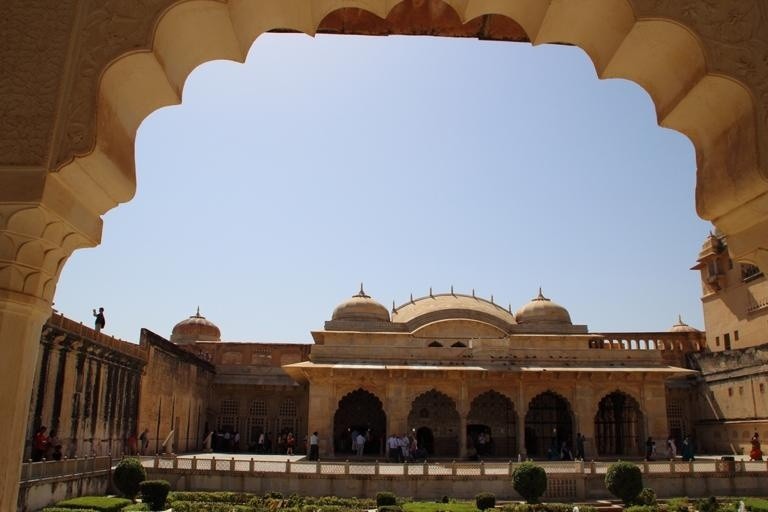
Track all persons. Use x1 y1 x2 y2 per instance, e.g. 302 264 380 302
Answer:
32 426 49 461
128 431 138 455
380 431 386 456
257 430 297 456
387 431 428 463
574 433 585 461
647 433 696 461
43 429 62 460
140 428 151 455
467 430 491 461
749 431 762 461
356 432 365 456
93 307 105 333
304 433 308 455
352 429 359 455
212 429 241 453
309 431 320 461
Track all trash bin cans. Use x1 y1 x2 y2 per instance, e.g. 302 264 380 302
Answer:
721 457 735 473
